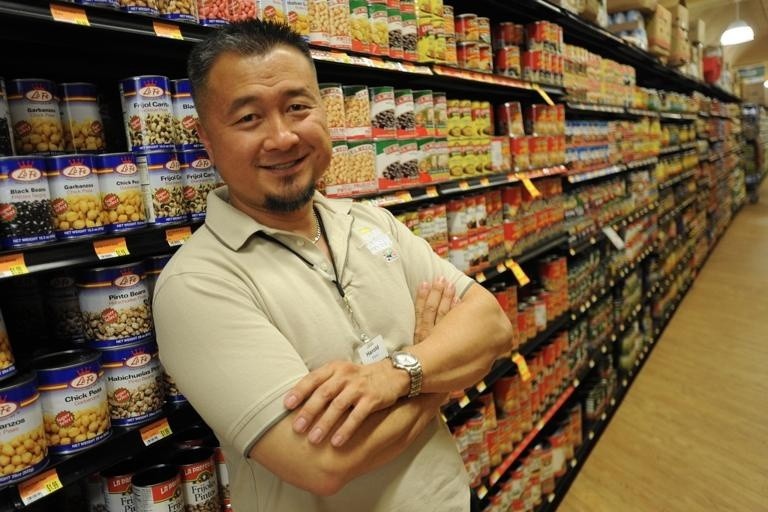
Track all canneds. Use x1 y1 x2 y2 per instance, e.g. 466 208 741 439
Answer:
1 0 565 512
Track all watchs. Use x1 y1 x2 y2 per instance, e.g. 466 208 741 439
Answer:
382 349 424 400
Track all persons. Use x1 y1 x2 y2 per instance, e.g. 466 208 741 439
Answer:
150 15 515 511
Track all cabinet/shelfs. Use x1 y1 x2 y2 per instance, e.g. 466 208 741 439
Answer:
0 0 745 512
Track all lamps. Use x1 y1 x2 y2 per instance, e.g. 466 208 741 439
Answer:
716 0 756 48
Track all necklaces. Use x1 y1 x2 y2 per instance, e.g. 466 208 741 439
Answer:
306 207 322 245
